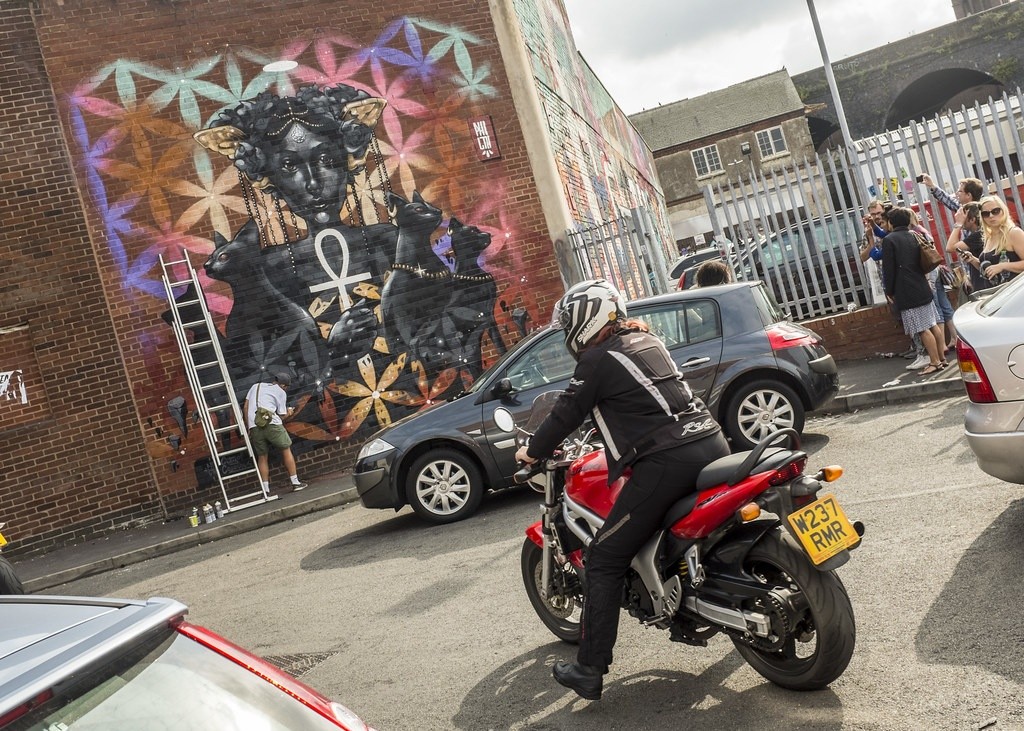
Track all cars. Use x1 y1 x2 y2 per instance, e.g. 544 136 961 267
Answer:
351 281 858 524
950 270 1024 482
669 239 789 294
0 585 376 731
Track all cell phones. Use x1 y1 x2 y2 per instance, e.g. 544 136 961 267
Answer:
916 175 923 183
956 247 969 259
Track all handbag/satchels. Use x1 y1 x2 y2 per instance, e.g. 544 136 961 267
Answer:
939 266 955 285
909 229 944 275
253 405 273 428
859 255 889 310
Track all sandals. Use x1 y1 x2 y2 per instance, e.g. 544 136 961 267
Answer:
939 359 949 367
917 363 944 376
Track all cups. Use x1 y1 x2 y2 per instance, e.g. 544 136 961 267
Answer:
189 516 198 527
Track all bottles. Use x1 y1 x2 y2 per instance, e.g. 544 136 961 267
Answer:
214 501 224 518
202 505 213 524
206 503 217 522
192 506 201 524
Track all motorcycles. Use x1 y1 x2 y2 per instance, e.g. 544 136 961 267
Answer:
482 386 875 693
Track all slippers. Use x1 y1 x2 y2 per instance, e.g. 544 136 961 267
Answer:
943 349 951 355
947 344 956 352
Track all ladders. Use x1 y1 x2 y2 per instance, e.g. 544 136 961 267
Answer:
158 248 282 517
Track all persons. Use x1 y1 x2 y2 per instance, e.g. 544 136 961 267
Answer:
514 276 730 701
859 200 960 376
242 372 309 497
678 261 731 341
917 172 1024 293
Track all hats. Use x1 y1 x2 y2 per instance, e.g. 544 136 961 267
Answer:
275 371 292 385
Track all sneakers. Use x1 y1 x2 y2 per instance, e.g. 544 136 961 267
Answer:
261 491 278 499
552 661 608 701
905 354 932 371
290 482 308 492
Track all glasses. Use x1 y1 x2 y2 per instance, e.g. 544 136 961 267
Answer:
871 211 881 218
981 207 1002 218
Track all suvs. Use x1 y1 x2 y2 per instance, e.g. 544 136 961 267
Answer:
678 201 937 326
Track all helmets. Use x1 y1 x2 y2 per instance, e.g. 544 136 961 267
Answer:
557 279 628 362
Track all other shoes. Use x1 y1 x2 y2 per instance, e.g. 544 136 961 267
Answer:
904 351 918 360
898 348 912 357
